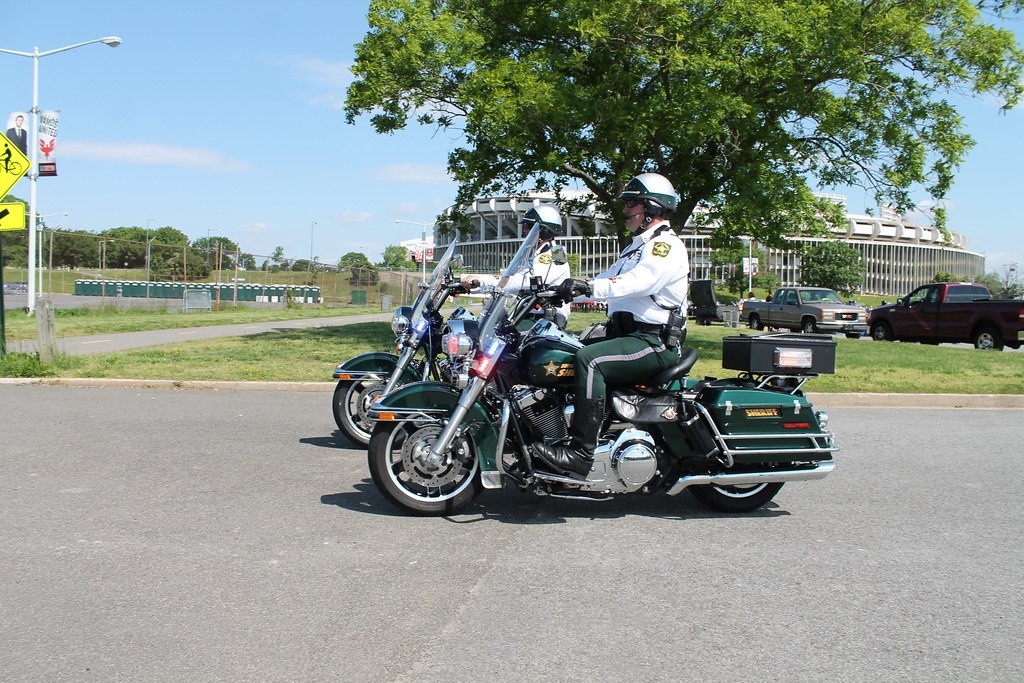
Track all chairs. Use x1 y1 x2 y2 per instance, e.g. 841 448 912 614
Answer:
788 293 796 300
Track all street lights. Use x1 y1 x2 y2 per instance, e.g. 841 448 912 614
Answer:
98 240 114 273
207 228 221 264
0 37 121 320
146 219 159 273
395 220 454 283
25 213 68 296
311 221 318 284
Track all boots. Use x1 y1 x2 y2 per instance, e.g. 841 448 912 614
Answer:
530 397 606 483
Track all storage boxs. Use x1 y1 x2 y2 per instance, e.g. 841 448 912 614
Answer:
721 336 838 375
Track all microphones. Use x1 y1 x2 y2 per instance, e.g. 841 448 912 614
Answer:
522 234 527 238
623 210 646 221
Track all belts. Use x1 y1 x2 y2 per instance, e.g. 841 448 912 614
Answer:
632 321 686 340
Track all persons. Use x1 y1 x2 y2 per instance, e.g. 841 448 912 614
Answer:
548 173 690 481
5 114 27 158
460 204 571 332
735 290 772 329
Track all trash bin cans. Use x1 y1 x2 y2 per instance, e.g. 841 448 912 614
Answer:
689 280 740 329
381 294 394 312
352 291 366 305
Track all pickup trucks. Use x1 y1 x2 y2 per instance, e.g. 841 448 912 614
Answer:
742 288 869 339
866 282 1024 352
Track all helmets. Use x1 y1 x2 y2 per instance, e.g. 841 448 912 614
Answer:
617 173 676 213
516 205 562 236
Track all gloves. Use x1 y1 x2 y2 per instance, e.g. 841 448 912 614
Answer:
555 278 592 304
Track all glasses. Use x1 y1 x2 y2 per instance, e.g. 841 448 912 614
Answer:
524 223 534 230
624 199 647 207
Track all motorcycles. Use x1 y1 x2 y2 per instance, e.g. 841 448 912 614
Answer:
366 223 840 515
333 239 606 449
